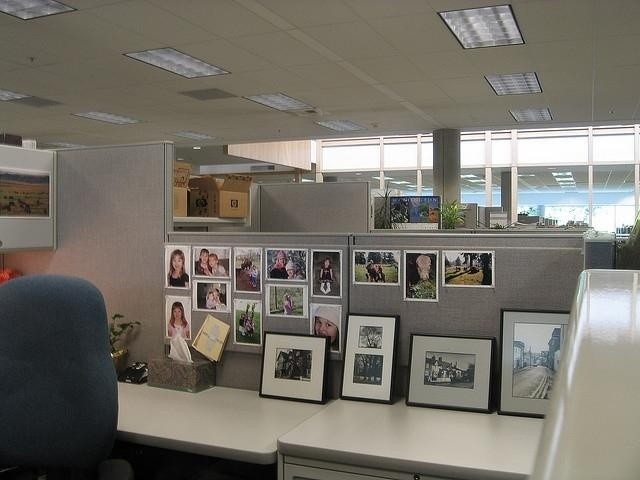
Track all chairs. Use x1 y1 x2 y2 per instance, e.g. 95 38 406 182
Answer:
0 275 119 480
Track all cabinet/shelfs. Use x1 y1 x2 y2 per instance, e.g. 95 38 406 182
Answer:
276 398 543 480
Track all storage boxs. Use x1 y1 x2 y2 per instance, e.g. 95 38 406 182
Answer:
173 161 192 216
188 173 253 219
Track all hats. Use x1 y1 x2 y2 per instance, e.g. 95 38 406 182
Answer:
276 250 297 272
315 307 339 330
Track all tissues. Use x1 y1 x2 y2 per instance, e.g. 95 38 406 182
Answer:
147 325 217 393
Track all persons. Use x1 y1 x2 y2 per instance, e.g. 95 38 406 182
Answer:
167 249 385 377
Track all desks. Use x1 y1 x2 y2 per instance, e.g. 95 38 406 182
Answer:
115 379 336 465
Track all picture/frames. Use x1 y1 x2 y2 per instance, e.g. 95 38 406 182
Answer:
497 308 569 419
260 331 331 404
0 167 51 219
352 249 495 302
164 244 341 354
340 313 399 404
405 333 495 414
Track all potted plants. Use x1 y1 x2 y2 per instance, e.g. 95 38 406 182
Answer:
108 314 141 379
